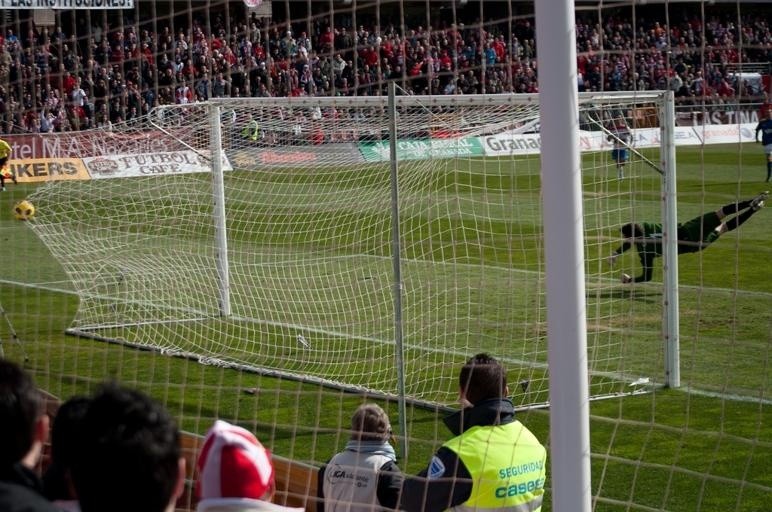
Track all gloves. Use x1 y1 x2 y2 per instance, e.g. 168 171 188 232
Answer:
607 251 619 266
621 274 632 284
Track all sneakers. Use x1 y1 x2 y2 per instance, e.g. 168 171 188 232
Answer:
764 177 771 183
616 174 625 179
750 190 769 213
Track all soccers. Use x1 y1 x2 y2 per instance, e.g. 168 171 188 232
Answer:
13 201 34 221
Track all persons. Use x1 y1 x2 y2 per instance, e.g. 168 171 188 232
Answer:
0 140 20 192
602 186 771 284
33 368 188 511
187 416 308 509
316 399 400 512
1 22 772 135
0 351 62 511
394 347 547 511
604 109 634 181
752 108 771 184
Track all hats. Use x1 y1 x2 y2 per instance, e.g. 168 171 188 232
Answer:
193 419 276 501
